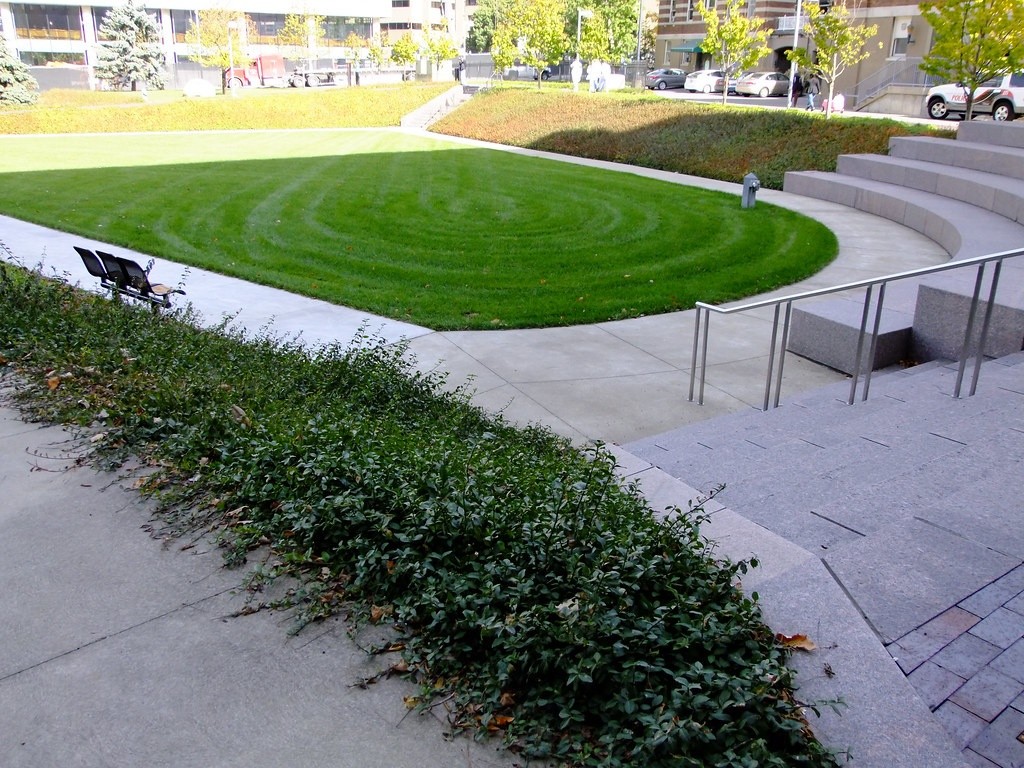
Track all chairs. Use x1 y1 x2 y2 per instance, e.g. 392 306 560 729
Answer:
74 244 185 315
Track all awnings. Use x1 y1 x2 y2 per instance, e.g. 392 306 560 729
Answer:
670 40 712 54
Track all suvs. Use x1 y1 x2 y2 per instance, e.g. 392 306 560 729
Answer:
925 69 1024 122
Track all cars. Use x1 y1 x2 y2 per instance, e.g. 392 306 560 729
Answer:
502 57 552 81
735 72 791 98
685 69 733 94
645 68 689 90
715 71 753 96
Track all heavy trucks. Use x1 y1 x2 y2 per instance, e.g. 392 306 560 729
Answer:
223 52 336 89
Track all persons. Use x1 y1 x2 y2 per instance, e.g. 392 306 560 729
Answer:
791 74 822 111
458 58 467 84
570 56 583 95
831 91 846 111
585 58 611 92
